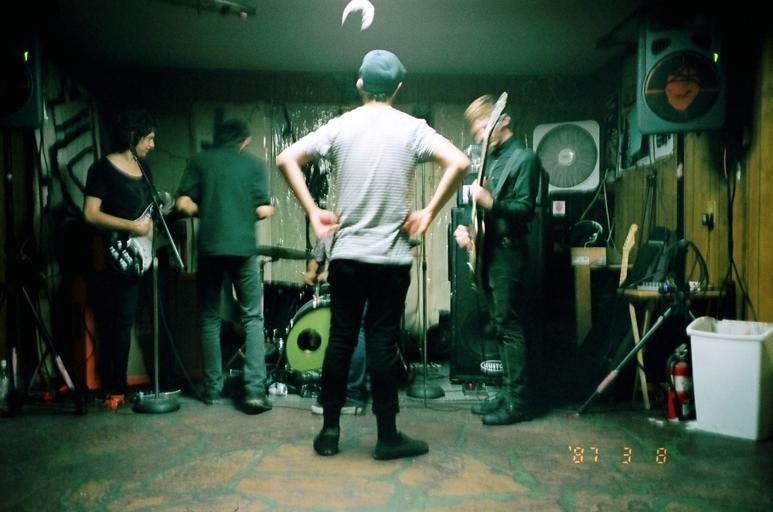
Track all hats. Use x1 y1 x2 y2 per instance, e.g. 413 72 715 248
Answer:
359 50 406 93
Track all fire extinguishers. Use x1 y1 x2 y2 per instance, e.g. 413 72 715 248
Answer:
665 343 692 420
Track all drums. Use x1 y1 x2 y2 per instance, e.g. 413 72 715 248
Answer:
262 280 295 338
280 281 366 389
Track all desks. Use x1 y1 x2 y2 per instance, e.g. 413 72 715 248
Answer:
615 287 726 411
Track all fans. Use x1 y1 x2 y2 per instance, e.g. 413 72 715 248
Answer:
533 120 602 193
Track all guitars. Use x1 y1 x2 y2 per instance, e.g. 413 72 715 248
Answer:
467 90 508 288
109 196 181 281
616 223 640 290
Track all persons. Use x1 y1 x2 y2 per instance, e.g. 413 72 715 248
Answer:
274 46 474 462
451 92 543 426
173 116 277 411
301 234 371 417
80 104 197 412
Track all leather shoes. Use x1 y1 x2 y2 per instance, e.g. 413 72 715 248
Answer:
472 394 531 425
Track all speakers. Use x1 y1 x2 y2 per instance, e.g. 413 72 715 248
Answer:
0 0 45 130
638 0 728 135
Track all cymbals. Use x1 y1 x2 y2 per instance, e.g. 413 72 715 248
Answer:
256 244 316 261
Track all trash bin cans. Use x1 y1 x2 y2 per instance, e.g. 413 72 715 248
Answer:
686 316 773 441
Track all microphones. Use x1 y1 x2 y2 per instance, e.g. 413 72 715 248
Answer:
128 128 138 154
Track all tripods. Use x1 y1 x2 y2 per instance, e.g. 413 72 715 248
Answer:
578 140 698 416
0 131 87 416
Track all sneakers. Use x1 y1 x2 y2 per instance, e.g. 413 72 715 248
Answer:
244 392 272 409
314 433 338 455
374 432 429 460
203 394 219 404
